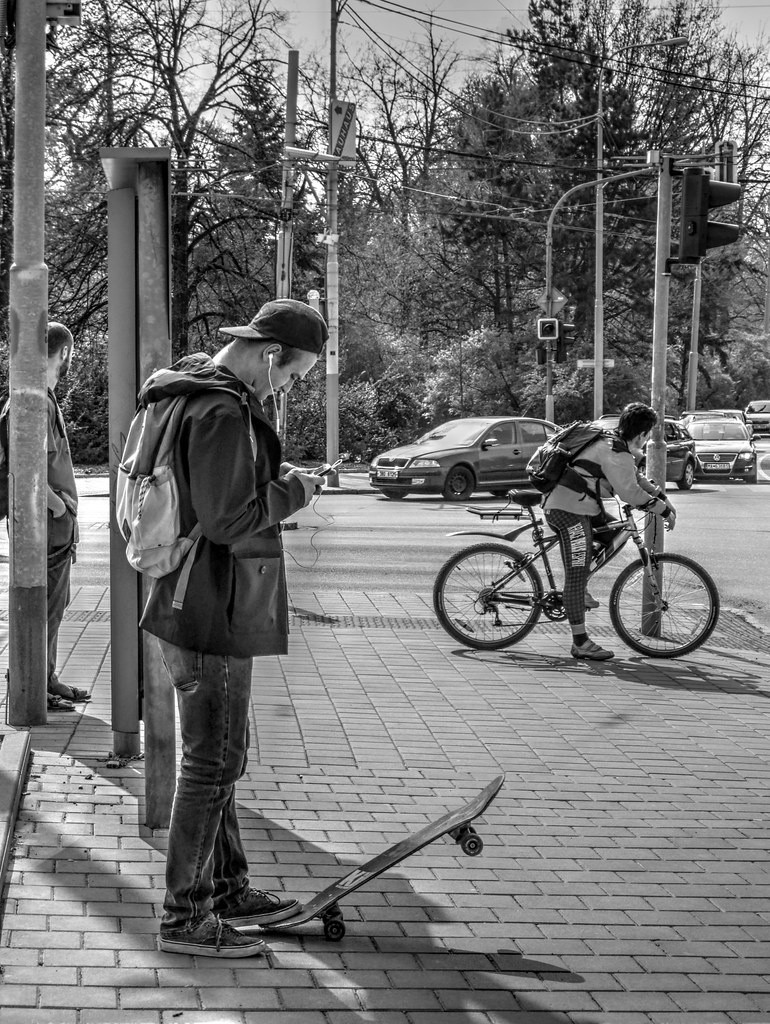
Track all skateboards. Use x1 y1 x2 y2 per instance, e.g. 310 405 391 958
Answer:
265 774 507 942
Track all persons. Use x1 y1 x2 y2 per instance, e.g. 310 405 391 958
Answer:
115 299 326 958
0 322 90 712
542 402 675 660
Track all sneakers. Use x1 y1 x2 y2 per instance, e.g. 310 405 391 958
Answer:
216 887 303 926
584 589 599 608
157 911 265 958
571 640 614 660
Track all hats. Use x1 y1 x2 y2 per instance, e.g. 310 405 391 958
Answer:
218 298 329 355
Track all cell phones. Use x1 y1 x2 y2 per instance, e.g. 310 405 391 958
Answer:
309 464 331 476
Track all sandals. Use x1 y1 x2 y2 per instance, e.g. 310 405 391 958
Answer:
47 691 76 711
48 681 91 703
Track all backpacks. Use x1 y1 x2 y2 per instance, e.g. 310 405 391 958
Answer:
525 421 632 501
0 388 65 521
115 380 257 610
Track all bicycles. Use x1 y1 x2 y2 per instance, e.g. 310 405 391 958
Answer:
432 489 720 660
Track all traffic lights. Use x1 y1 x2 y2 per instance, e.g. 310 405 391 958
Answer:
538 318 559 340
681 166 741 259
556 321 575 363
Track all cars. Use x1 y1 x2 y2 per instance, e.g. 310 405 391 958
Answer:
680 409 754 441
368 417 566 501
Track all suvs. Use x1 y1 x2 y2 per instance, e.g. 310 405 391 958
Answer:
685 416 761 484
590 415 697 490
745 400 770 430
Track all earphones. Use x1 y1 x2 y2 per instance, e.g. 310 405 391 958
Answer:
268 354 274 368
